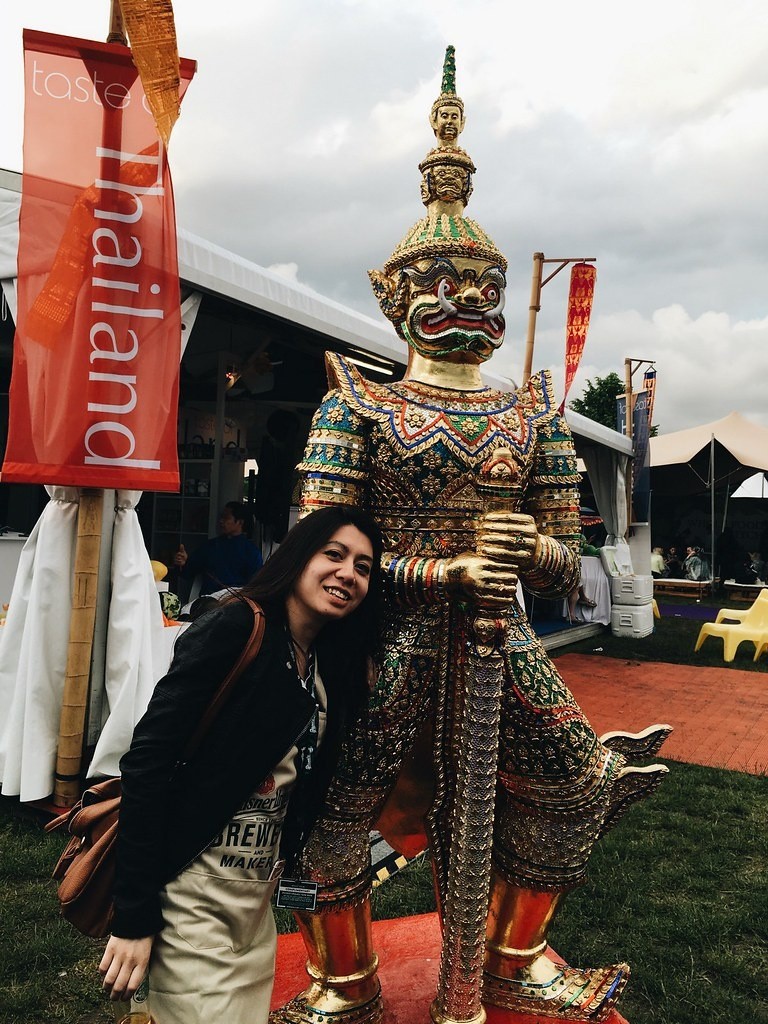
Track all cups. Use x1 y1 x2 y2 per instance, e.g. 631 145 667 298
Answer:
197 478 210 497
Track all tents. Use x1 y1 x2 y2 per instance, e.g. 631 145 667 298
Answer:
575 410 768 578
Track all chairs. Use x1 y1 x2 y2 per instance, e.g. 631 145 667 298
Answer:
715 588 768 652
695 599 768 662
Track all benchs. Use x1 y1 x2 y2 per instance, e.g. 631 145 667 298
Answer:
722 580 768 601
654 574 720 599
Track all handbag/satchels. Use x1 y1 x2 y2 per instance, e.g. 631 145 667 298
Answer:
43 775 122 939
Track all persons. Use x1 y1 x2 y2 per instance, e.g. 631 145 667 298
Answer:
267 46 630 1024
99 503 379 1024
651 546 711 593
175 501 263 596
744 553 766 583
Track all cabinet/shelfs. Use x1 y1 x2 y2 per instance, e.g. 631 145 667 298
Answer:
152 458 245 601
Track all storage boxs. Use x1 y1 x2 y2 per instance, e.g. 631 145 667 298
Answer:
612 605 654 638
600 546 653 605
